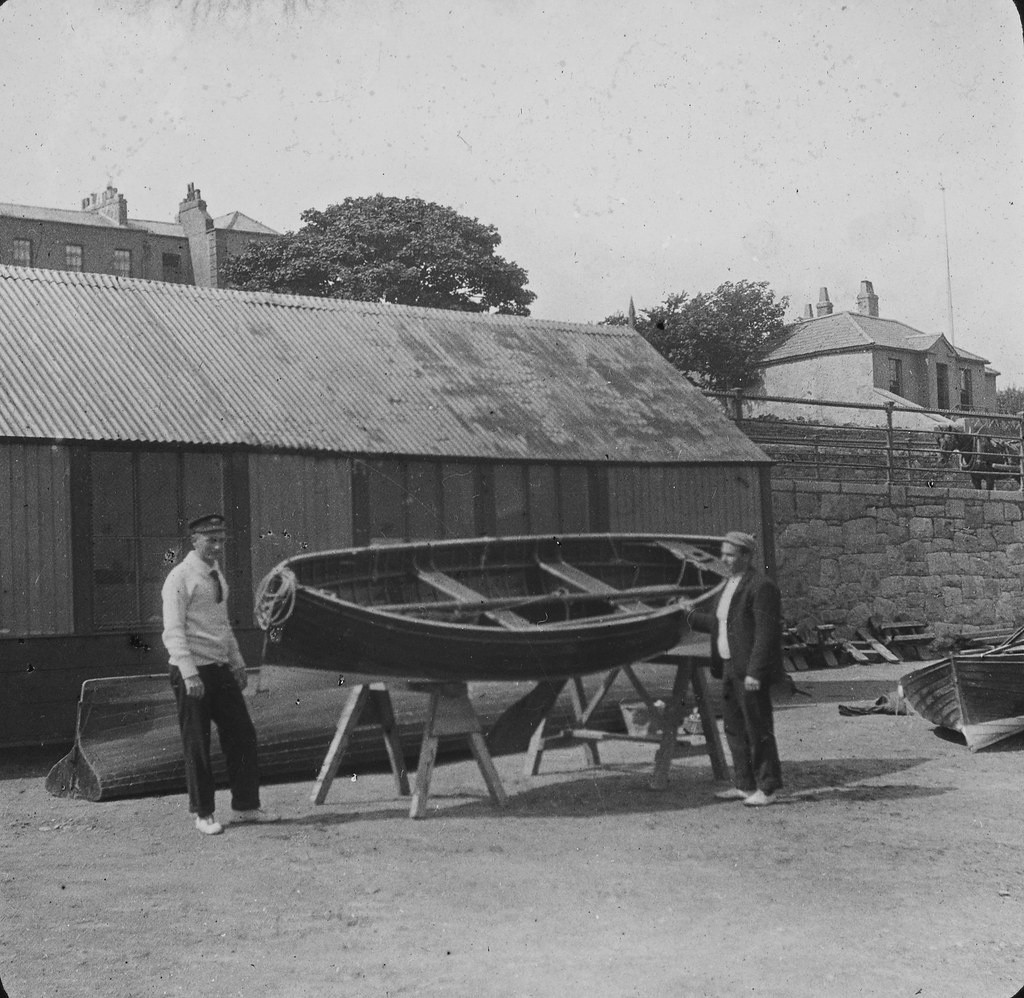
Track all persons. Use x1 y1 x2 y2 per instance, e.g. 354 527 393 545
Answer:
159 512 282 837
677 530 784 808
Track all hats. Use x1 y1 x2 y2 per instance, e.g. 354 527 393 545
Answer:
188 514 229 532
722 531 759 551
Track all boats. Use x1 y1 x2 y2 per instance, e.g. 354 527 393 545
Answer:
896 636 1024 760
254 529 740 690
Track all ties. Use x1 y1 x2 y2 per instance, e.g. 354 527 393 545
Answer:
208 570 223 603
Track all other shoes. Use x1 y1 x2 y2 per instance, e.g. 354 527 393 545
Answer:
195 815 223 834
229 808 281 823
714 787 757 798
743 788 790 805
